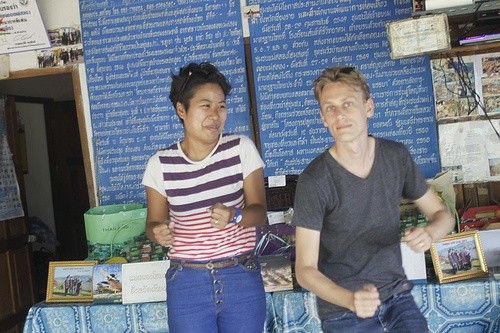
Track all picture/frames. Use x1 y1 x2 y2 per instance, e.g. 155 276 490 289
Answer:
430 229 490 286
45 260 98 303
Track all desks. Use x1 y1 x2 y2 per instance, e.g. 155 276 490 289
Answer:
30 266 500 333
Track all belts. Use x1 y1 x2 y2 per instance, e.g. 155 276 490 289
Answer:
170 255 250 269
395 282 414 294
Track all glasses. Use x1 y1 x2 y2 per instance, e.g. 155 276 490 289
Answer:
339 67 368 94
174 63 222 104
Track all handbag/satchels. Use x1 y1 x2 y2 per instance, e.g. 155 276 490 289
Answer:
426 169 456 215
84 203 164 261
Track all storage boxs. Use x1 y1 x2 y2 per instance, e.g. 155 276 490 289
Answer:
398 240 427 285
385 10 452 61
458 205 500 230
258 253 293 293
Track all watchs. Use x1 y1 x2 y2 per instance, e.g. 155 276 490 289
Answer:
228 207 242 224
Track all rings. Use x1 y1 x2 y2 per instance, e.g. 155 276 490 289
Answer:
215 220 218 224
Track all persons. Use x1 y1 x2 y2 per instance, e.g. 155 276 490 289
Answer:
448 247 472 274
61 48 79 62
65 276 82 296
141 62 267 333
292 67 457 333
38 52 58 68
63 27 81 44
